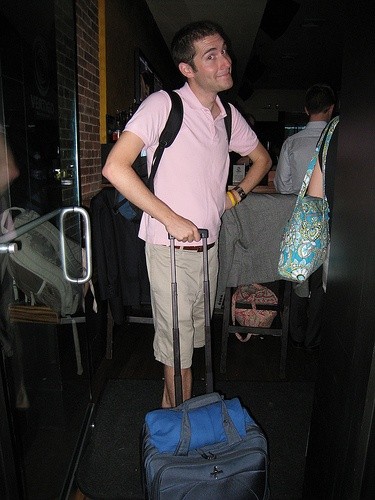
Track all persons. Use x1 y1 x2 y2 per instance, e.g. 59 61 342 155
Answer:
102 22 272 409
274 85 341 354
0 115 21 244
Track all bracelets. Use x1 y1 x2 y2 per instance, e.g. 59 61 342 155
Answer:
227 190 238 206
233 186 247 202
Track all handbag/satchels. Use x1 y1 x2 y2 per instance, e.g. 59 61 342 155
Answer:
230 282 279 342
277 115 330 284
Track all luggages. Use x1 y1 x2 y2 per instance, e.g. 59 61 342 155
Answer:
143 226 267 500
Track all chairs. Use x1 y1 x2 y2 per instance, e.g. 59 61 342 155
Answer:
89 184 155 360
213 193 321 376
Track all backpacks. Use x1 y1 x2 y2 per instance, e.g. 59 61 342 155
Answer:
0 207 83 316
109 88 233 222
